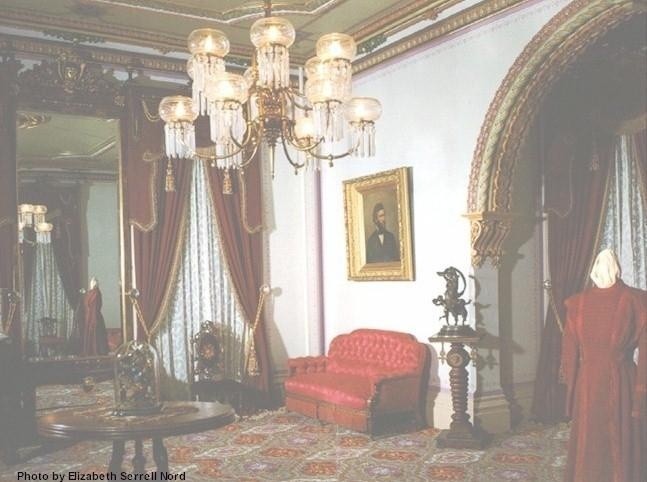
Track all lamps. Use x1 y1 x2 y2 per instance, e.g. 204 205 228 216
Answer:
140 1 382 176
17 203 54 245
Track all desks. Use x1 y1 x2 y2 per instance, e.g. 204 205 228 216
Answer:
34 397 237 481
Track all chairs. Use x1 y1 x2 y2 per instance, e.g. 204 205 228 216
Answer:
189 318 247 413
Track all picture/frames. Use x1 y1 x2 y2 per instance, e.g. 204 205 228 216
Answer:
342 166 414 281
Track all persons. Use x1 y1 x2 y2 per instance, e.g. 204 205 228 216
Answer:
559 248 647 482
80 277 111 356
365 202 399 263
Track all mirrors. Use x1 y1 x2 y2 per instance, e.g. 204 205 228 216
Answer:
0 48 130 367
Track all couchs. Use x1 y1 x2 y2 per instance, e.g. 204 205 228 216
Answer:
281 327 432 438
